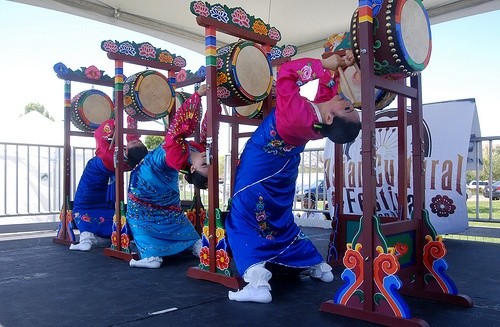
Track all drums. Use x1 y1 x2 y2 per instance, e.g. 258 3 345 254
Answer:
232 78 276 119
216 42 273 107
123 70 173 121
341 49 395 110
70 90 114 133
162 92 203 127
350 0 432 80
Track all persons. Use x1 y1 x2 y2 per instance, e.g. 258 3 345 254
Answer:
127 85 223 268
69 117 139 251
296 193 325 220
228 49 361 302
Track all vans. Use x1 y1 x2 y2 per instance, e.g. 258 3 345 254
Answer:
469 179 494 190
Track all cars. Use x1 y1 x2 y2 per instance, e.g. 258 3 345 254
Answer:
483 180 500 200
296 179 327 202
